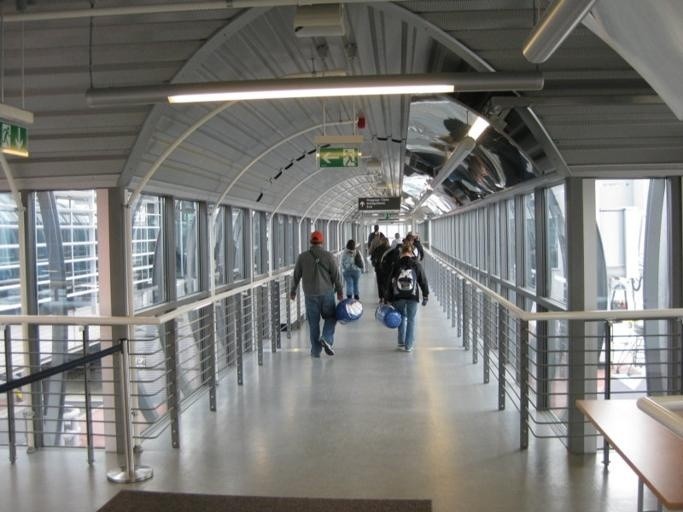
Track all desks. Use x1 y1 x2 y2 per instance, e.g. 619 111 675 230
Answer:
573 390 682 511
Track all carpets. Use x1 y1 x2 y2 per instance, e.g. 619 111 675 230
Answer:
93 489 432 512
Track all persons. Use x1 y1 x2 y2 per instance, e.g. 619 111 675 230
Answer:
290 225 428 358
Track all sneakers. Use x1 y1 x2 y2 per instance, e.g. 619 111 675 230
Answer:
395 344 417 353
310 338 335 358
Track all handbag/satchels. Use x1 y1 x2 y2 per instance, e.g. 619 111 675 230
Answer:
334 297 363 320
375 304 402 329
354 254 363 267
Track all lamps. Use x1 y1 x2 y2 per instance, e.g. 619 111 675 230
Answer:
84 0 543 108
518 0 598 65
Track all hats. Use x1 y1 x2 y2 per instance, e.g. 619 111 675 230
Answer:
308 230 325 244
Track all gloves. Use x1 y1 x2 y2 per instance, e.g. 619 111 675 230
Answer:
335 292 343 299
421 296 429 306
288 292 297 302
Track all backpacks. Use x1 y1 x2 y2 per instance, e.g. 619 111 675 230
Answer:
392 259 416 296
